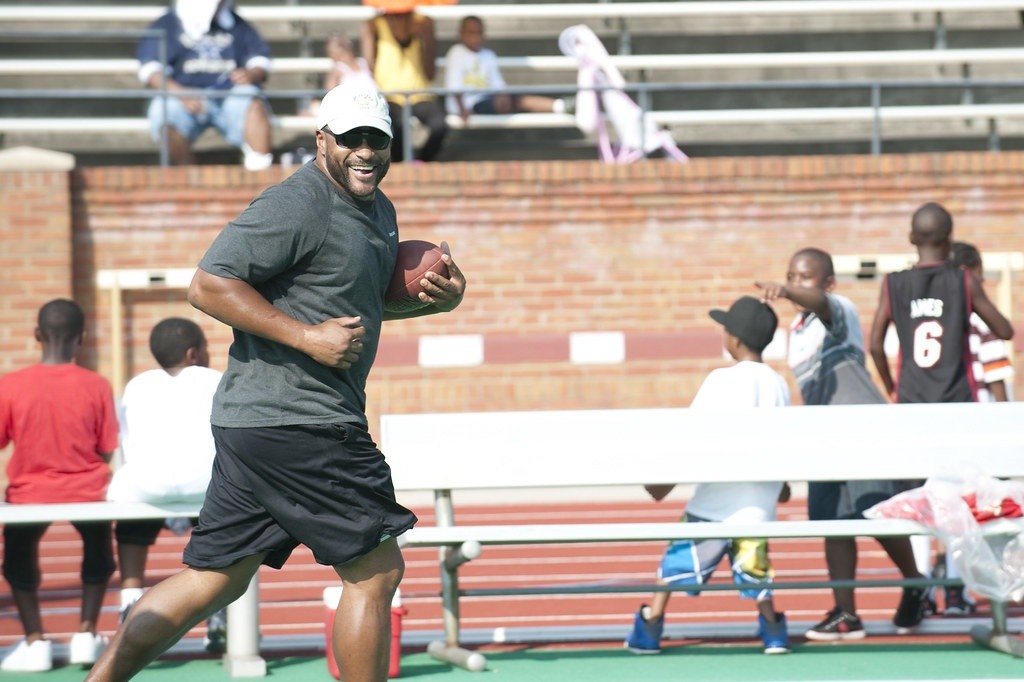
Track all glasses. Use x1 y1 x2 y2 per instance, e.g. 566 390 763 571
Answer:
323 128 389 149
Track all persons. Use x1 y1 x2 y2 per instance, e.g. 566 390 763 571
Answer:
444 16 577 121
624 295 792 653
0 298 117 672
870 201 1016 613
137 0 272 166
84 83 466 682
107 316 225 653
301 0 450 163
753 247 928 639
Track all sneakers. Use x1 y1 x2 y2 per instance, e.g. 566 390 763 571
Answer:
759 611 791 653
804 607 867 640
625 603 664 655
943 597 975 616
923 598 936 615
70 632 109 663
2 638 53 671
893 587 929 636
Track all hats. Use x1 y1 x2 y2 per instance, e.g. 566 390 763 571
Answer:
709 297 777 350
318 83 393 139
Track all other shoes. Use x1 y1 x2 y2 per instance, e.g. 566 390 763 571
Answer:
206 614 226 654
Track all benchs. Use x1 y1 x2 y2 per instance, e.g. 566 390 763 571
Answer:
0 0 1024 162
0 497 268 677
375 405 1024 672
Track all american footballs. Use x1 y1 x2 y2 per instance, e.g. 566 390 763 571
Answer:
384 240 449 314
